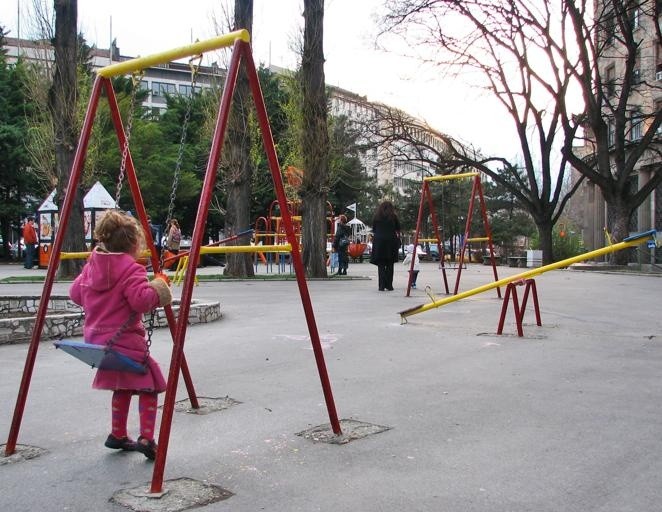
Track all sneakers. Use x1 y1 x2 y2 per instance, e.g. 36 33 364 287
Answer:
135 436 158 459
105 434 136 451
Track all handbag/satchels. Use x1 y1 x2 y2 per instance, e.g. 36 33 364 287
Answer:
339 239 350 246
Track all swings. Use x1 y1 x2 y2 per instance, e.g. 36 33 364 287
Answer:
438 178 467 270
52 54 203 376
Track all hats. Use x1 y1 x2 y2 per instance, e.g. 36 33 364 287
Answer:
407 244 414 253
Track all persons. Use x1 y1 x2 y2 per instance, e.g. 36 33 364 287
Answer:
168 219 182 271
403 244 420 288
23 217 37 269
148 216 160 247
371 202 400 291
70 211 171 460
333 215 352 275
